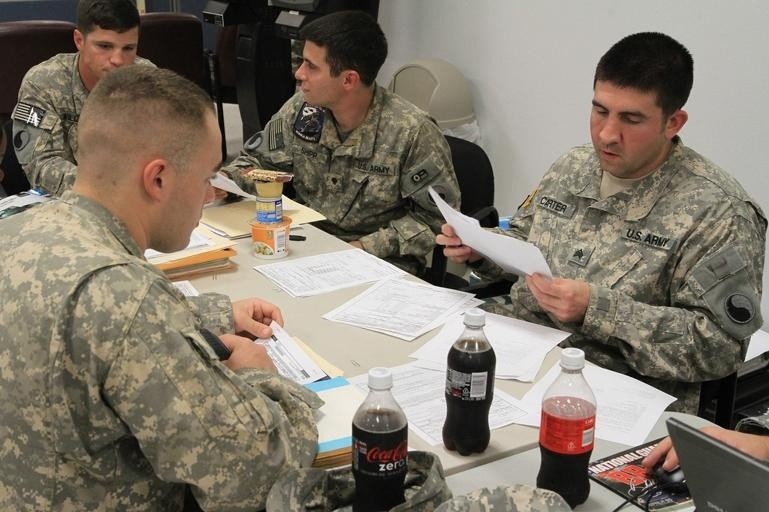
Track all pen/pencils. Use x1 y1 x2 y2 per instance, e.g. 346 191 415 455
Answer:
288 235 306 241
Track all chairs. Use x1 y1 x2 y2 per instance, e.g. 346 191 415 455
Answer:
699 371 738 431
0 18 77 194
134 13 228 165
420 135 520 299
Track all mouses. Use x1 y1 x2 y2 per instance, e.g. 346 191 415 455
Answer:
653 459 688 494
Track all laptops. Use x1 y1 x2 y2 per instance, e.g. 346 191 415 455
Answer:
666 417 769 512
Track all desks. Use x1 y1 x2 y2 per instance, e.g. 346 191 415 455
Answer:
444 410 727 511
143 189 586 480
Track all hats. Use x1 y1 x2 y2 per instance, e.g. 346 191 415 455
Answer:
433 483 573 512
264 448 454 512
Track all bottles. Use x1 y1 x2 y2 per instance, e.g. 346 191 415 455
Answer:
535 346 600 512
348 366 411 512
440 308 497 457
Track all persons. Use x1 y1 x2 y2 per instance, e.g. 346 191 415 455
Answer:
213 9 463 278
10 0 159 197
640 407 769 472
435 30 767 414
0 63 325 512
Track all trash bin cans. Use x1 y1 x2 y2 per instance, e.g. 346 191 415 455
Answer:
388 58 482 147
